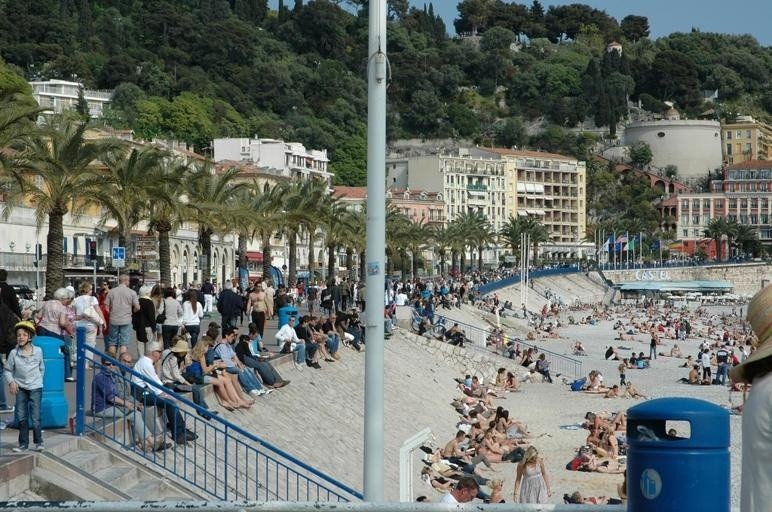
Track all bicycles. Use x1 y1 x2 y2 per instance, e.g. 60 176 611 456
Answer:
409 315 449 339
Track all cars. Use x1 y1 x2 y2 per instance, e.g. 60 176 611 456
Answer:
10 285 36 301
391 275 402 282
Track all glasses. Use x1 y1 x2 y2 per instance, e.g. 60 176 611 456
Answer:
104 362 115 367
289 319 295 322
156 351 162 354
103 283 108 286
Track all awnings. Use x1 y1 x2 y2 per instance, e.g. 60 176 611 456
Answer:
236 251 274 260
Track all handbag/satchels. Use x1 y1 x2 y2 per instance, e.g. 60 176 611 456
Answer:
83 306 104 326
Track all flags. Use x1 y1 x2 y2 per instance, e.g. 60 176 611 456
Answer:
596 229 660 255
668 239 682 252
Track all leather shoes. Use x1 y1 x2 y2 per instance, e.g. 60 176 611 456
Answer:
157 427 198 451
202 411 218 419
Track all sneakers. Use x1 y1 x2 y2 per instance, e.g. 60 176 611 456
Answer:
13 445 28 451
294 358 322 372
250 380 291 396
36 442 45 450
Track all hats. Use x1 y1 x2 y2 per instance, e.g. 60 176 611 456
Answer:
208 322 221 329
170 341 191 353
731 282 771 384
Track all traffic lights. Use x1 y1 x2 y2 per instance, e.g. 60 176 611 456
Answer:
89 241 98 261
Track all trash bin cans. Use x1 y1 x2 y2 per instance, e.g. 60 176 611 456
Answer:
278 306 299 330
421 291 429 299
625 397 731 512
14 335 69 430
441 288 449 296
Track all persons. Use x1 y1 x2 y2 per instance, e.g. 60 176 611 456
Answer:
384 264 521 318
529 263 577 272
1 269 365 453
654 254 744 267
729 285 771 512
530 277 566 305
418 298 759 504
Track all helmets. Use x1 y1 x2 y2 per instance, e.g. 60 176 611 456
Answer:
13 320 36 337
